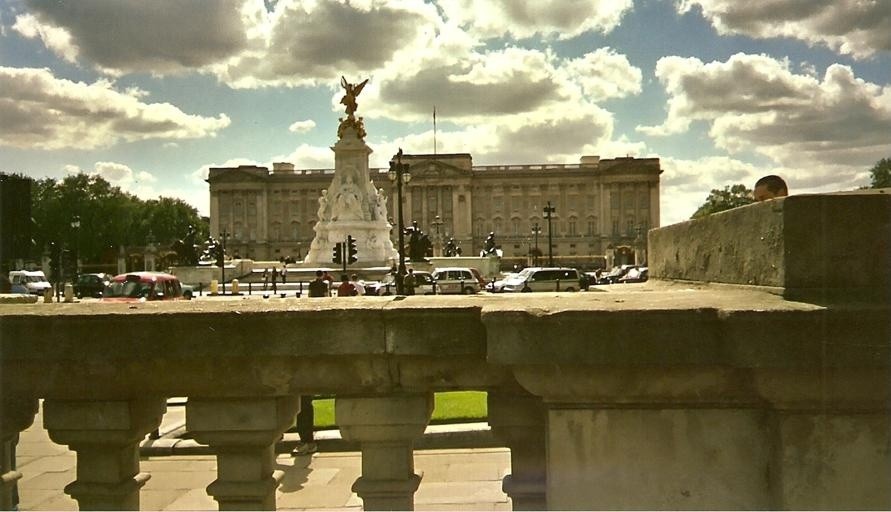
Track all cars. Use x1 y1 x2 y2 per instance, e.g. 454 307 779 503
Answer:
74 273 115 300
97 270 195 303
597 263 649 285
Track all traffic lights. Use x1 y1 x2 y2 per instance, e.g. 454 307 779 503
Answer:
333 242 342 265
348 234 357 264
215 251 223 267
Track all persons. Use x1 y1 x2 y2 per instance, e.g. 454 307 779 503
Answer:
404 222 499 257
316 175 388 221
753 175 787 201
184 225 233 265
263 265 420 295
292 397 318 458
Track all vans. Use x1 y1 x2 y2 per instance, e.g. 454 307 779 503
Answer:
7 269 53 296
363 266 586 296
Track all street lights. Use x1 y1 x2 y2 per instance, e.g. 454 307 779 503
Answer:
70 214 82 275
387 147 412 297
542 201 559 266
530 223 542 267
218 228 232 256
431 216 444 233
